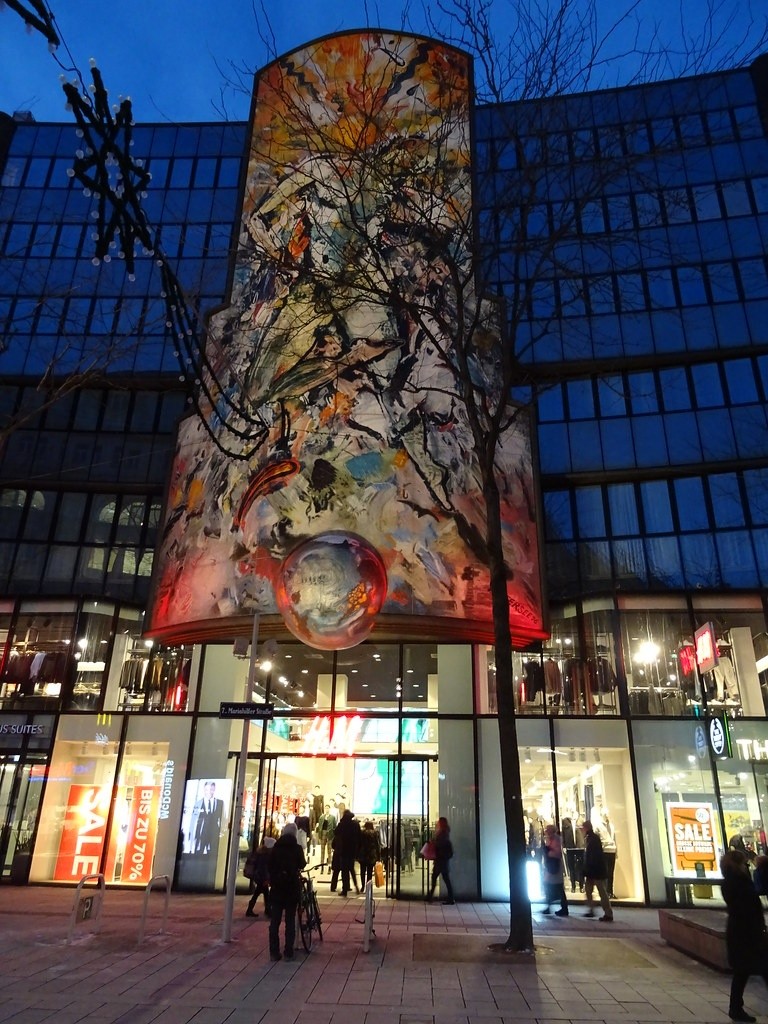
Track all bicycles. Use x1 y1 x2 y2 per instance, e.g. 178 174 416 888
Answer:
298 863 328 955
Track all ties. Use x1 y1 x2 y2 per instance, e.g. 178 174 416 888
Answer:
208 800 211 814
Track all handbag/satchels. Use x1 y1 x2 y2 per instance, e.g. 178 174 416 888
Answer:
373 861 385 887
546 857 561 875
421 842 435 861
243 853 256 880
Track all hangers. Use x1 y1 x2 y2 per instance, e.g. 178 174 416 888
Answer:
17 647 67 653
124 655 159 662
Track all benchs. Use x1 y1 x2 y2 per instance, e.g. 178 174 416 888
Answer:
665 875 724 902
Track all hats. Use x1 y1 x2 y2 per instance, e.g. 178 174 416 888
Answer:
364 821 374 830
282 822 297 836
263 836 276 849
342 809 354 820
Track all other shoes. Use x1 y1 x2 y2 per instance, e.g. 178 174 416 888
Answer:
283 950 293 960
338 890 347 896
541 908 551 914
729 1008 757 1024
245 910 259 918
554 909 569 916
581 912 596 917
599 915 613 921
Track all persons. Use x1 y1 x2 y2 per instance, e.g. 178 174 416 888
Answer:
330 809 380 897
243 784 428 876
539 825 569 916
189 783 224 854
529 808 621 900
265 823 307 965
421 817 457 905
245 836 276 918
720 850 768 1024
582 820 615 922
